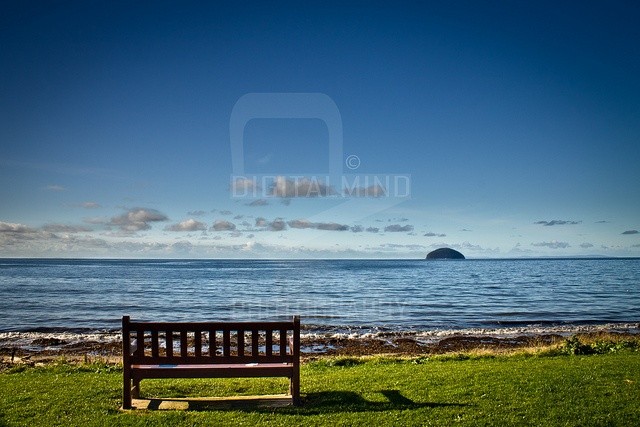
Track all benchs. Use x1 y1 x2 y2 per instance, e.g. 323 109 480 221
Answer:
121 313 301 406
